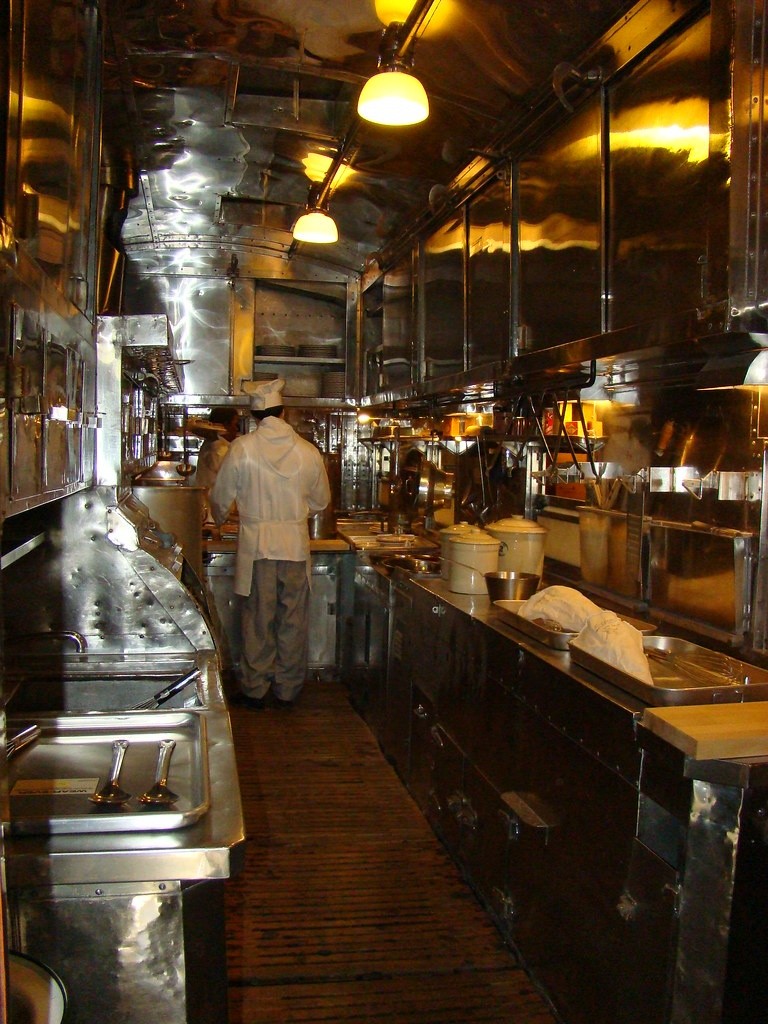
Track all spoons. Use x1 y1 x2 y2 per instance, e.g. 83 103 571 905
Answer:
88 740 132 806
138 740 180 808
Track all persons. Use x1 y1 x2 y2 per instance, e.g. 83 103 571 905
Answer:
196 408 240 533
211 378 332 712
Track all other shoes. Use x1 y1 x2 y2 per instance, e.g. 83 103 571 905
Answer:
274 699 294 709
228 692 265 712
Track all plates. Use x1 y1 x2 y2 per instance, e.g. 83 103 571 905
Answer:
253 372 278 382
298 345 337 358
262 344 297 356
321 371 345 398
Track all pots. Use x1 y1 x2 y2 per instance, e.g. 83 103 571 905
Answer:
437 555 541 606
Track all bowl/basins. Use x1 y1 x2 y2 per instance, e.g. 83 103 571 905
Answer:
382 557 439 578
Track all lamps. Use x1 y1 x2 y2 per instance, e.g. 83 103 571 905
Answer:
292 182 339 244
356 22 429 126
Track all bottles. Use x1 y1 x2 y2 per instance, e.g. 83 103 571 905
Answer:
439 515 548 594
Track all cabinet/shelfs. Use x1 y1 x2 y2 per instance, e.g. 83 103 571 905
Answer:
0 0 103 519
345 0 768 406
201 553 768 1024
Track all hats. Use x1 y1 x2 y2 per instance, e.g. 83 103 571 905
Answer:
240 378 285 410
